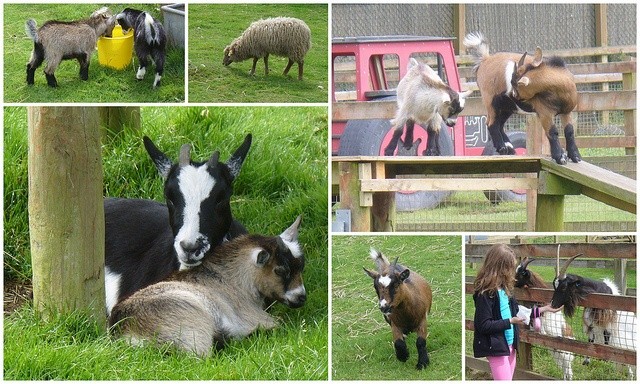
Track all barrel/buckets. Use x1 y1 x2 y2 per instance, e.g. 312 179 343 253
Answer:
96 25 135 70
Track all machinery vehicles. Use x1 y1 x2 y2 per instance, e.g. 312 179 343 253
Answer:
332 35 527 211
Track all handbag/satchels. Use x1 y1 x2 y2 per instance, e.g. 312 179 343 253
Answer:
533 306 541 332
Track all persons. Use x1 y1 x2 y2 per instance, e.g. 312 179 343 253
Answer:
473 244 565 380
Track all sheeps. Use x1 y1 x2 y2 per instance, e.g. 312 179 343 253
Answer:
463 32 583 163
223 16 310 82
550 255 638 378
361 246 432 369
513 255 575 381
383 59 473 159
25 7 115 88
117 7 167 89
102 132 254 318
110 218 306 358
